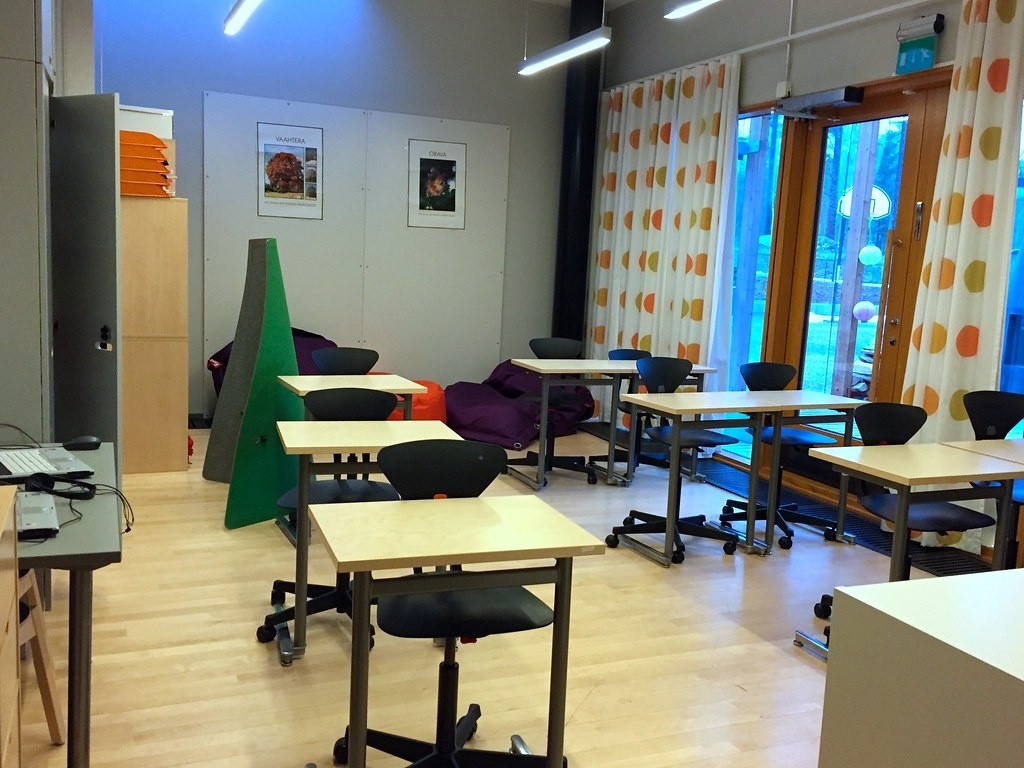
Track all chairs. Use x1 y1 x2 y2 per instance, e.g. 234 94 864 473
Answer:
258 336 1024 768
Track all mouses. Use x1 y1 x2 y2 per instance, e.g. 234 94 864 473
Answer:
63 435 101 451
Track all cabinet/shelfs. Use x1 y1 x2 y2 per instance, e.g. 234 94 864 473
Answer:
0 485 23 768
119 195 189 475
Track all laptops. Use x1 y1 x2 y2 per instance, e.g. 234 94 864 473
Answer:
0 447 95 486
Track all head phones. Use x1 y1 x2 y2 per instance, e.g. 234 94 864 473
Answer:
25 472 96 500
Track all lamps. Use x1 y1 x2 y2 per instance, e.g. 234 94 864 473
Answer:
664 0 718 19
223 0 260 36
515 0 611 76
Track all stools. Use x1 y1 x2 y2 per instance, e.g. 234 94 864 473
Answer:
18 568 66 745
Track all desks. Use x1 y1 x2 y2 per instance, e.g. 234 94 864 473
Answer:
618 391 782 569
0 443 121 768
276 374 428 550
794 444 1024 659
507 359 633 492
590 360 718 487
710 389 873 555
307 494 606 768
941 438 1024 464
274 420 466 667
818 567 1024 768
